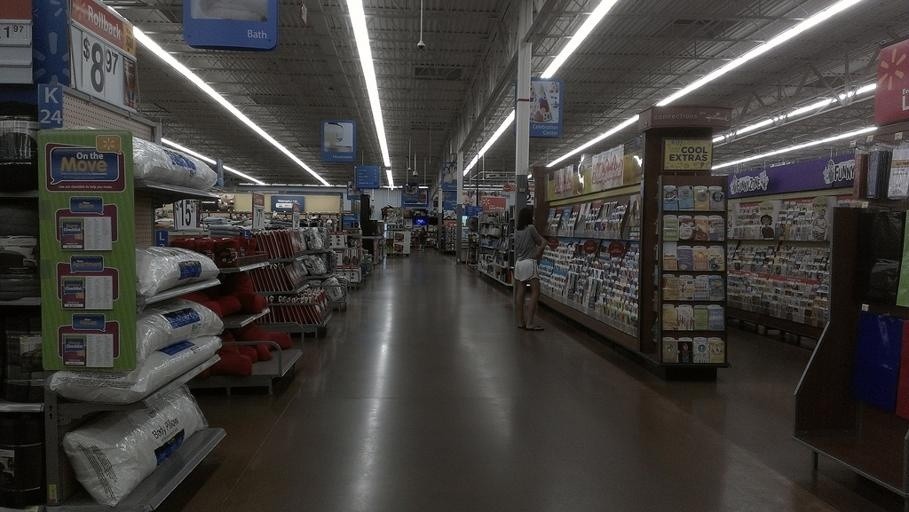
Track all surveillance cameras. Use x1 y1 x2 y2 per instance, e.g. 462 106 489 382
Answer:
418 41 425 49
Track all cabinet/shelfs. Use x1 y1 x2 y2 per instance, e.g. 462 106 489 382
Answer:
478 130 865 375
0 83 366 512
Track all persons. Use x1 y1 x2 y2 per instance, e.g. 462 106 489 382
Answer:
513 205 544 330
